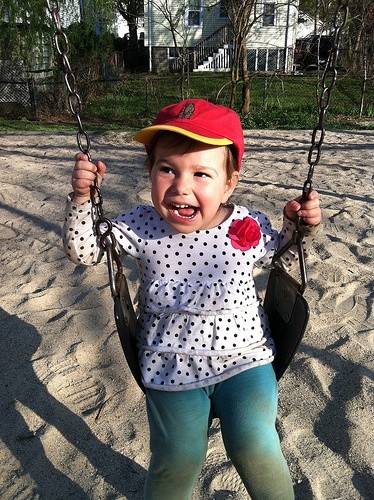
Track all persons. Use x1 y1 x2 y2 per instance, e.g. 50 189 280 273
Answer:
62 96 323 500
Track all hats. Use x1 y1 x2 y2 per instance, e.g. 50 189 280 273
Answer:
135 98 244 171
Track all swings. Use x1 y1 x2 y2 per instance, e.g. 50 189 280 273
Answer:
43 0 349 416
